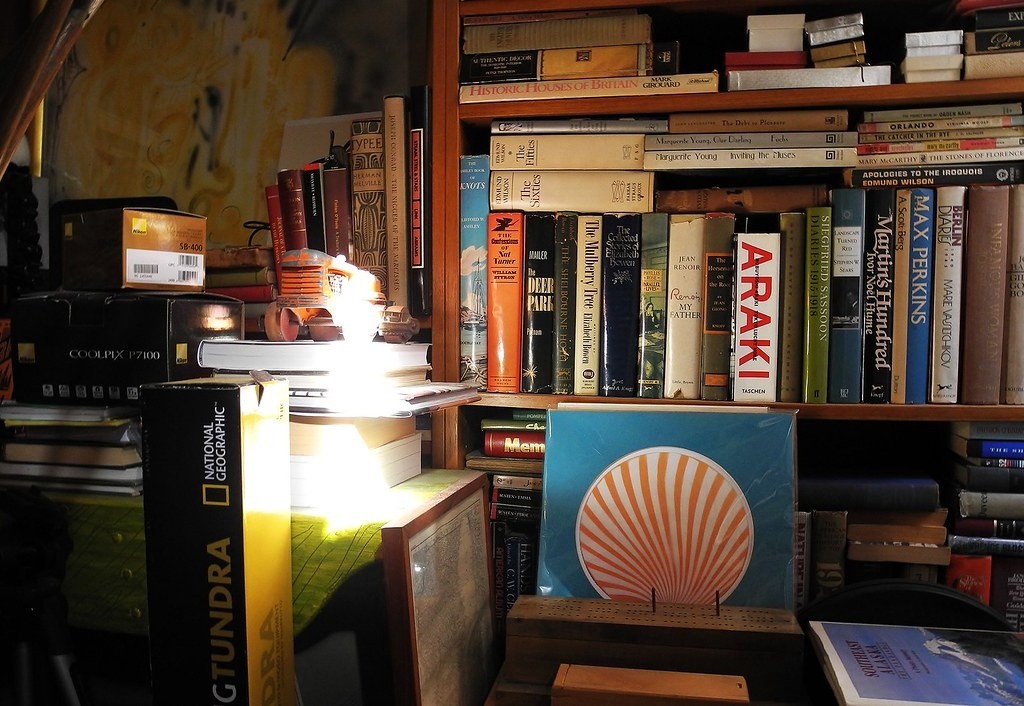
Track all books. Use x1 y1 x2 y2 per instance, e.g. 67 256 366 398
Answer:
206 246 276 332
0 403 144 495
458 0 1024 104
845 102 1023 405
459 109 868 403
265 82 431 315
196 339 482 510
465 398 1024 705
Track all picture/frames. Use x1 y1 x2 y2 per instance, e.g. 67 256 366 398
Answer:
381 470 499 706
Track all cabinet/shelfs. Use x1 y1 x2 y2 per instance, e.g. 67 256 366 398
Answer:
430 1 1024 654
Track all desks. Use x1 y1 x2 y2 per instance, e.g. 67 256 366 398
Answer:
0 472 470 706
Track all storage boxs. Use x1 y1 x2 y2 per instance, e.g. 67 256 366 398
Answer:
12 286 246 408
58 205 207 293
287 415 420 499
724 0 1024 90
140 373 297 706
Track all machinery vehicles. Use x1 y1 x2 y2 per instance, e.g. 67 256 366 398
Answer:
265 248 418 344
